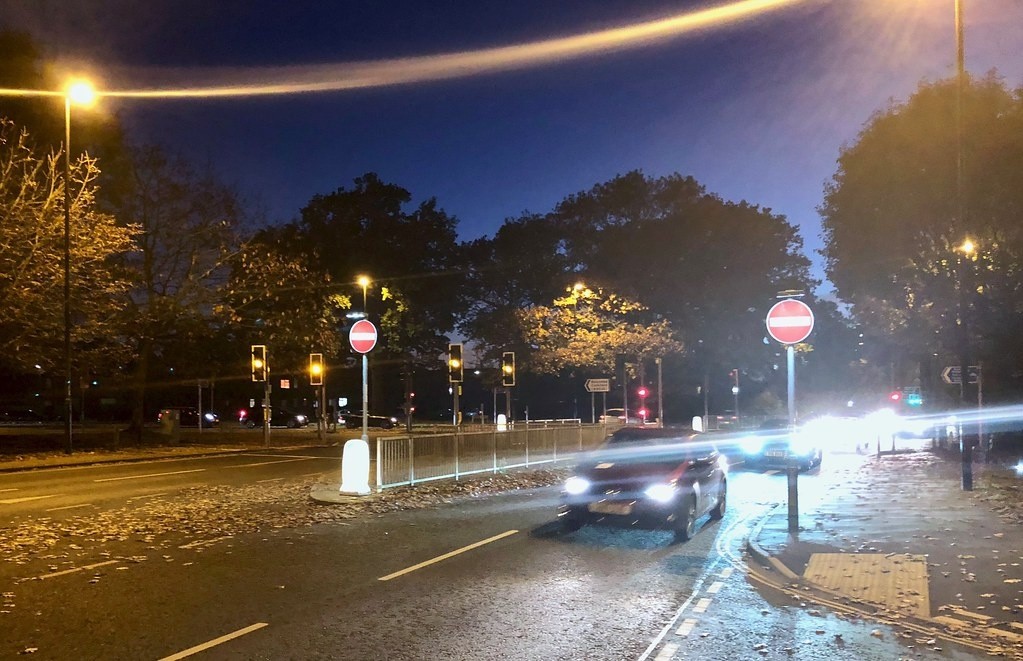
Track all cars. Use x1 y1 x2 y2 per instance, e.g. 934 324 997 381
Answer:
741 416 822 470
157 406 220 428
337 408 399 430
554 428 729 541
598 407 643 424
239 405 309 429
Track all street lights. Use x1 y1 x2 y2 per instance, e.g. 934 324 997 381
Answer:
732 368 739 417
654 357 664 428
60 77 101 456
356 274 370 312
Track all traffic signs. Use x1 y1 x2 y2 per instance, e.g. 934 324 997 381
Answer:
941 365 980 385
585 378 611 392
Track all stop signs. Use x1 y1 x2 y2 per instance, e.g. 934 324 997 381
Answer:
766 298 814 344
349 320 378 355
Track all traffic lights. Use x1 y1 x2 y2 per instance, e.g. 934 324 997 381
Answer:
448 344 463 383
251 344 267 383
309 353 323 385
501 351 516 387
890 391 902 403
638 408 649 418
637 388 649 397
